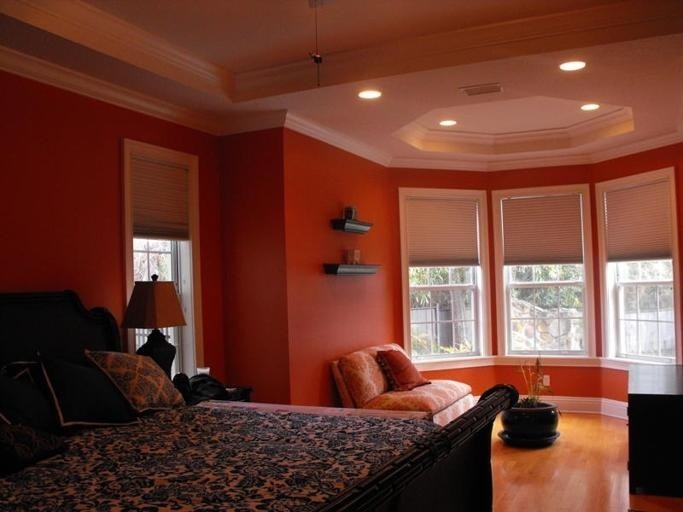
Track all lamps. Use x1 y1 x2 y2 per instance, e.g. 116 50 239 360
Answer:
120 275 187 382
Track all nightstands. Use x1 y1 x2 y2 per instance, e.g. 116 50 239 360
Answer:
212 385 254 402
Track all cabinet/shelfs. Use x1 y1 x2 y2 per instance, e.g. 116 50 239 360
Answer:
323 218 376 276
626 365 683 497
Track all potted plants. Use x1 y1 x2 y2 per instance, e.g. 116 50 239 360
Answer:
501 358 558 439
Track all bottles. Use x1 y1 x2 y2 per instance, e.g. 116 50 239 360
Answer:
344 208 356 220
197 367 211 376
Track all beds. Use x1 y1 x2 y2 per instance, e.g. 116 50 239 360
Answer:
0 291 518 512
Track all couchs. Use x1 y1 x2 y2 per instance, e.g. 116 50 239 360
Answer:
330 342 475 428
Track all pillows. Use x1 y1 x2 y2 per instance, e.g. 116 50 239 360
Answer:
376 347 431 392
36 350 141 428
82 349 187 414
0 422 72 476
0 359 63 442
